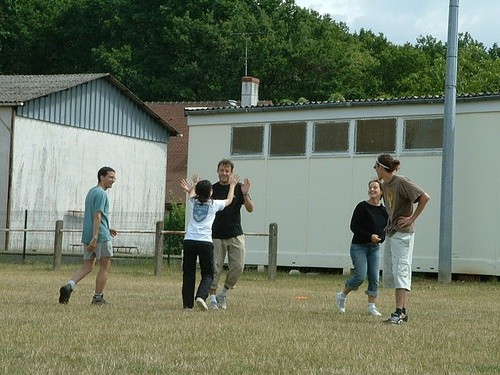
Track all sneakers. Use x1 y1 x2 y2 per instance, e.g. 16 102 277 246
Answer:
337 292 347 313
380 312 408 324
367 306 383 318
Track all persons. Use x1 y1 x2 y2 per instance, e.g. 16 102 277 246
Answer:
373 154 430 324
336 180 389 315
182 174 241 310
180 160 254 312
59 167 118 305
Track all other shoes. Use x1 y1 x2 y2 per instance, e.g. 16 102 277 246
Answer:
59 283 73 304
217 292 227 310
210 301 220 311
195 297 208 312
91 294 111 305
